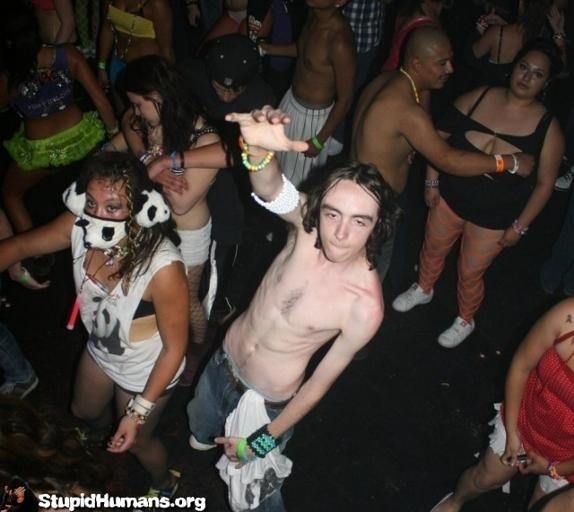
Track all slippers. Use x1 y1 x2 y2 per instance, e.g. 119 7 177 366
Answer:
428 491 461 511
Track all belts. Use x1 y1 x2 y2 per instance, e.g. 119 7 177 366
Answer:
216 335 300 411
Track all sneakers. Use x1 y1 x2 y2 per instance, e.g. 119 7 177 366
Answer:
144 476 178 510
1 373 42 405
436 314 477 351
392 283 434 314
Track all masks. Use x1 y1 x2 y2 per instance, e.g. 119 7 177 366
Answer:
78 210 135 251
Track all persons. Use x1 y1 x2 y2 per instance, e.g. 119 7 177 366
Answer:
1 1 573 511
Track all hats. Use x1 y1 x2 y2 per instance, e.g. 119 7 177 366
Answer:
210 31 261 89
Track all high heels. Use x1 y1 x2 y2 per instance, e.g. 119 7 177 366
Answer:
180 345 214 389
